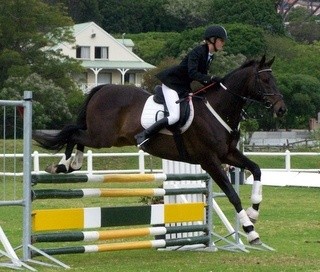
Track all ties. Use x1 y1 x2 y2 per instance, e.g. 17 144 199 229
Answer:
208 56 213 64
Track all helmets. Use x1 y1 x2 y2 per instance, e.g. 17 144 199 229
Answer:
204 25 228 40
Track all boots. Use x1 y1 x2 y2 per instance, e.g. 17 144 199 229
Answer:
134 117 169 150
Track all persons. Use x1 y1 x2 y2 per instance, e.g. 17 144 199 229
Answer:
133 25 227 153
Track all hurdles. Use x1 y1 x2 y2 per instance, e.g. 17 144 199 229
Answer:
0 90 276 269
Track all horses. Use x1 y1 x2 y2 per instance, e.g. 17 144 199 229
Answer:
32 52 287 244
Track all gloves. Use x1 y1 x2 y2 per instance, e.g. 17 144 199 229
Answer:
208 75 221 85
